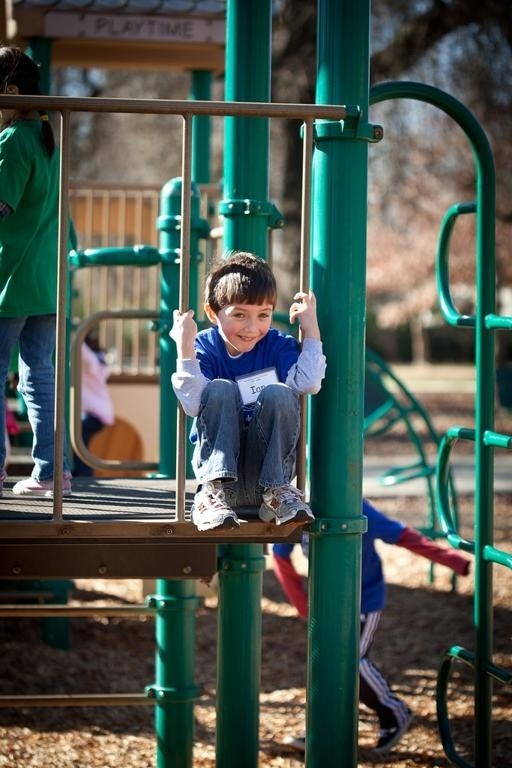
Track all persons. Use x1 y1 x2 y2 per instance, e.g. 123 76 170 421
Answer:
169 252 327 532
273 494 471 755
0 48 72 498
72 331 115 476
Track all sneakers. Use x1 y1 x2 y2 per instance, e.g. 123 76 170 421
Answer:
259 485 316 526
284 734 307 750
12 475 71 497
189 481 240 532
374 710 413 753
